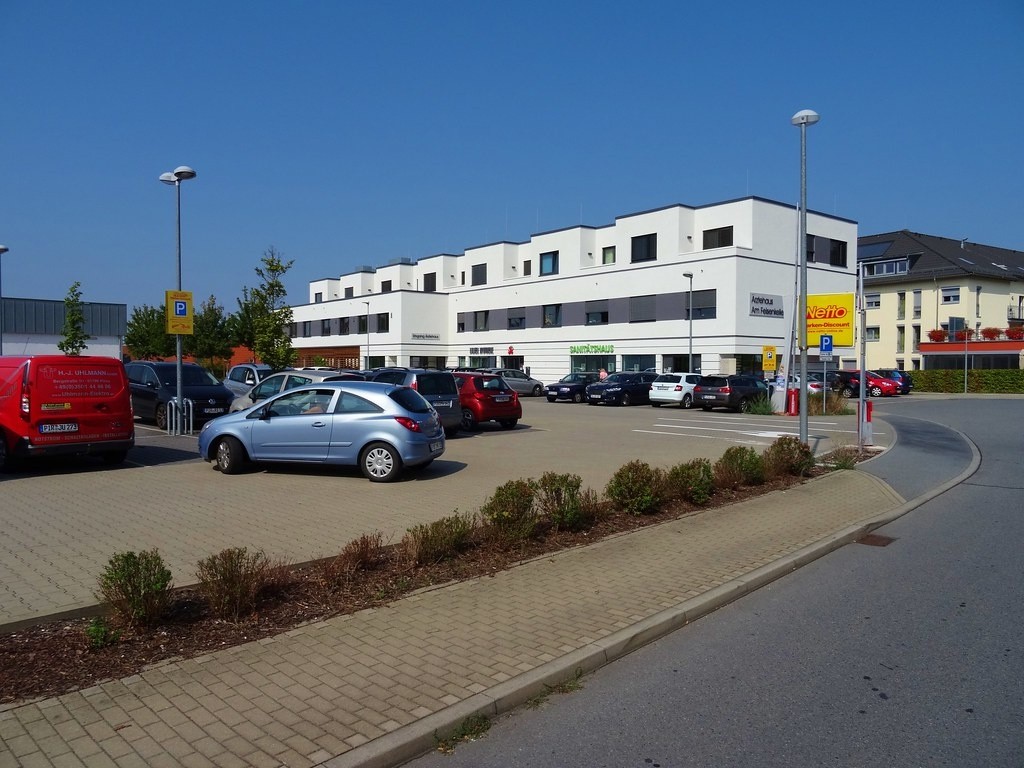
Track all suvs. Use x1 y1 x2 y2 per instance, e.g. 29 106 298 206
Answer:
224 363 304 398
368 365 462 436
450 371 523 431
649 373 703 410
586 372 658 406
227 370 368 414
124 360 235 429
475 368 544 397
544 372 601 404
693 373 767 411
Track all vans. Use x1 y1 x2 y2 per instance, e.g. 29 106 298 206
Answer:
0 350 137 472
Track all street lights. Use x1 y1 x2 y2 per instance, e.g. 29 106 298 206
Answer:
788 108 823 447
681 271 694 372
362 301 370 371
0 246 10 355
159 166 197 434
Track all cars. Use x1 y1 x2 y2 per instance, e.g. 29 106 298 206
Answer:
196 381 447 483
294 367 373 376
763 372 828 395
840 369 902 396
870 368 914 395
808 371 867 399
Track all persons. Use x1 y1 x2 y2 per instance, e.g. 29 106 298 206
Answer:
303 390 329 414
600 369 608 381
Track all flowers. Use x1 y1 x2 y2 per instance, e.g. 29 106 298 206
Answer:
955 328 975 341
980 327 1004 341
1006 327 1024 340
928 330 948 342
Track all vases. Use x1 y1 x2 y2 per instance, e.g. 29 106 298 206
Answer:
960 334 972 341
933 335 945 342
990 336 996 341
1009 335 1023 339
